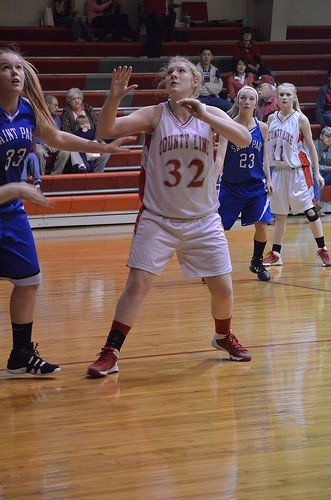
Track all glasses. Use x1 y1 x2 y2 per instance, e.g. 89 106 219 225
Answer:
322 133 331 138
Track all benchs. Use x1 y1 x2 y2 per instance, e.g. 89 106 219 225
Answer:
0 26 331 228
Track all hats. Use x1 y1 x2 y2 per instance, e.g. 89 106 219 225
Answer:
252 75 274 87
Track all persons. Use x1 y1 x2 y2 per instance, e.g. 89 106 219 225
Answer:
260 81 331 269
85 55 253 379
309 125 331 224
193 26 282 123
198 84 276 284
316 68 331 130
29 87 113 174
54 0 174 57
0 43 139 377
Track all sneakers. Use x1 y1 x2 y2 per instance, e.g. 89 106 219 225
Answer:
250 256 271 281
211 329 252 362
88 347 120 376
261 251 283 266
7 342 61 376
317 246 331 267
201 277 206 284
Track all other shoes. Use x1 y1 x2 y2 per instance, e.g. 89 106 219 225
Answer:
91 36 98 42
79 167 87 173
91 159 103 171
37 187 42 192
77 37 86 42
